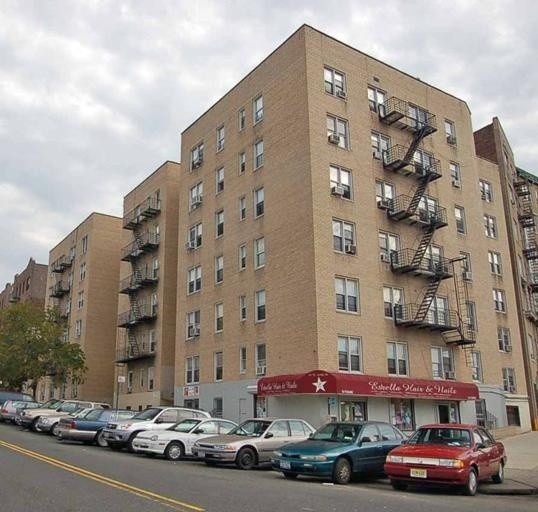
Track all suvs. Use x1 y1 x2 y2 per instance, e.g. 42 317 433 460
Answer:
0 391 113 436
101 404 211 453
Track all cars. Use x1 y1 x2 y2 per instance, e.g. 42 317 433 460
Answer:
270 419 410 485
384 422 508 496
190 416 318 470
55 406 142 447
130 416 252 461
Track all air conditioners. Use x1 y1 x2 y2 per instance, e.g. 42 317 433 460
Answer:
377 201 389 209
329 134 340 144
345 243 357 254
190 327 200 337
332 186 345 196
337 90 346 99
